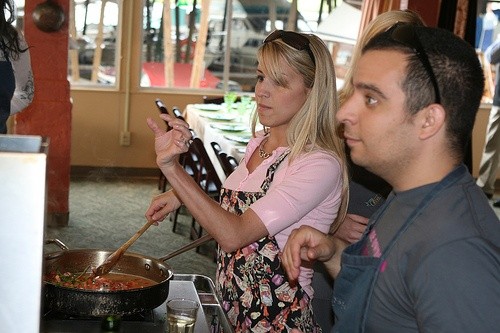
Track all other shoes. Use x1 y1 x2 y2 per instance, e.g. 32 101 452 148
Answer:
485 192 500 208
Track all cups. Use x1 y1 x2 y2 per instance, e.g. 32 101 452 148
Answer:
166 298 200 333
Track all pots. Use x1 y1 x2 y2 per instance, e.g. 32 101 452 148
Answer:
43 233 216 318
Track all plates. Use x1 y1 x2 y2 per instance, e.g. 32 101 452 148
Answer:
198 112 235 120
195 103 220 111
224 134 251 143
209 122 247 132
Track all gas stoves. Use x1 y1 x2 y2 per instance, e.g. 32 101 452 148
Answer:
40 274 235 333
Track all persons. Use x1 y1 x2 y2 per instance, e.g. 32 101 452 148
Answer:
309 9 426 333
473 34 500 210
0 1 34 135
146 29 350 333
279 21 500 333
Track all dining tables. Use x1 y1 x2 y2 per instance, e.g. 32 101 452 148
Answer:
182 98 273 183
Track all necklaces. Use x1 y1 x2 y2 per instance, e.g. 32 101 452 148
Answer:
258 140 277 159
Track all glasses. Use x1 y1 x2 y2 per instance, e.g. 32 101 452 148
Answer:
263 29 316 66
389 22 440 103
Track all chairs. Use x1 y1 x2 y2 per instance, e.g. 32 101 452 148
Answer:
155 99 239 264
